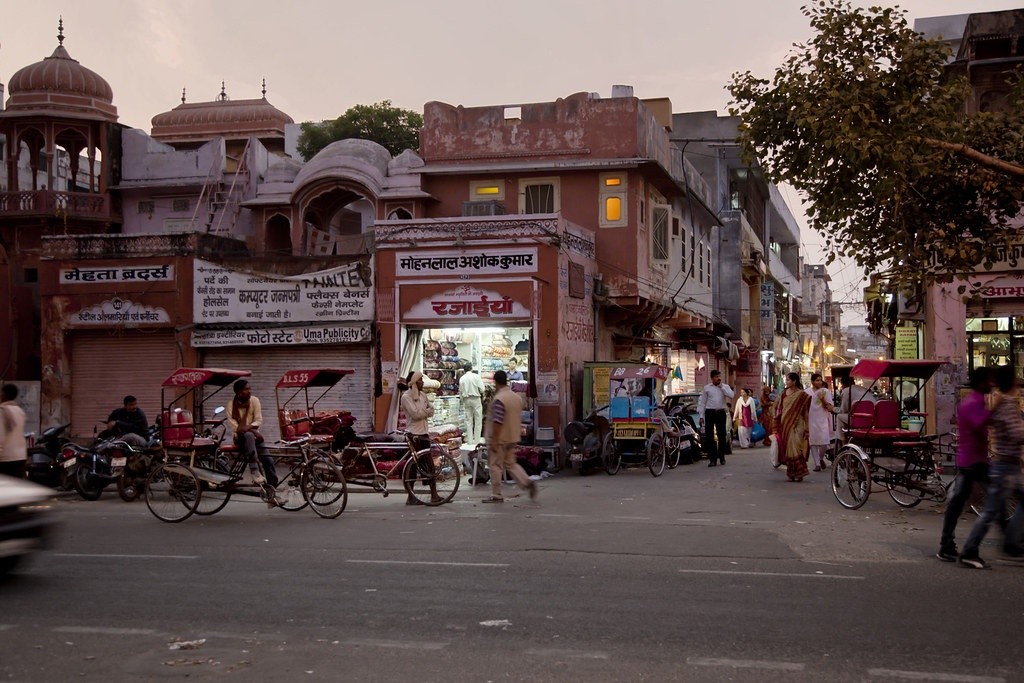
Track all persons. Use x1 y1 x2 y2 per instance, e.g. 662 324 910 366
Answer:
936 366 1024 569
98 396 149 440
226 379 288 511
698 370 881 482
459 358 538 503
0 384 26 478
401 372 455 504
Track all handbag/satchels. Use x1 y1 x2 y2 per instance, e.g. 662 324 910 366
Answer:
769 434 781 467
751 423 766 443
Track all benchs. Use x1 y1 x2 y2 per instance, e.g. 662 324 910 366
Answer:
277 408 334 445
162 410 215 448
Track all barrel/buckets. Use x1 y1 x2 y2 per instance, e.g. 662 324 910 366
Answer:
908 420 922 432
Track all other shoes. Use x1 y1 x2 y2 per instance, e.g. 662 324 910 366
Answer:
267 496 289 509
820 459 826 469
813 466 821 471
959 555 992 568
741 447 745 449
1003 543 1024 554
251 473 265 484
720 454 726 465
708 461 716 467
482 496 503 502
936 548 960 561
668 432 682 438
528 480 536 498
797 478 803 482
788 476 795 481
749 445 753 448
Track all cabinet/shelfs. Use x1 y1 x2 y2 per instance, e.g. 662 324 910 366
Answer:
422 367 460 399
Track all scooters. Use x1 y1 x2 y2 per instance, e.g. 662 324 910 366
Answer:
563 404 612 477
664 401 701 462
17 419 141 502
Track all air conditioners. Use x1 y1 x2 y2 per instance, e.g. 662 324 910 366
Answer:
776 318 789 335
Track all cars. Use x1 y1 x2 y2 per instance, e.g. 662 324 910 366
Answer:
0 469 63 576
661 392 734 455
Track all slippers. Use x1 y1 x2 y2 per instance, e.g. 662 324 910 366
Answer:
406 498 420 505
431 497 453 503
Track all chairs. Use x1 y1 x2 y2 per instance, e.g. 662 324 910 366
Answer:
848 399 920 440
611 395 660 421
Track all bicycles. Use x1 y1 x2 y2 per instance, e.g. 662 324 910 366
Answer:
335 429 462 505
268 435 349 519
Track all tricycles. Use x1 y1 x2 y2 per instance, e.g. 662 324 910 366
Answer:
830 359 943 512
141 368 317 521
582 362 680 476
275 365 353 476
